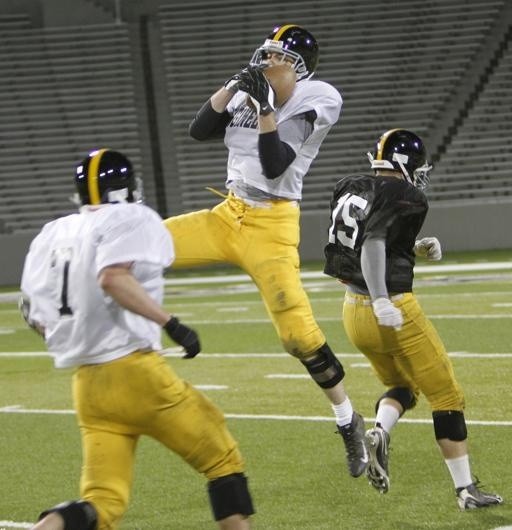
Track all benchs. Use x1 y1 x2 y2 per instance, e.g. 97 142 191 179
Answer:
1 3 143 238
0 1 512 239
158 2 512 213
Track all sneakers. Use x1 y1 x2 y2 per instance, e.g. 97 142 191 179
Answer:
335 411 369 479
453 483 505 511
364 426 391 496
18 297 46 339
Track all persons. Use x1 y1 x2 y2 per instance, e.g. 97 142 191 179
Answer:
19 146 256 530
159 24 369 480
323 129 506 511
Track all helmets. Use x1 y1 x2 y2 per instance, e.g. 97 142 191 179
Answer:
371 128 428 187
257 23 321 83
74 147 141 206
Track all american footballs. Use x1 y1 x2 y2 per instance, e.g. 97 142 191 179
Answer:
246 65 296 112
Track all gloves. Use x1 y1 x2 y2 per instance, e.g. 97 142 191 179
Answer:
223 65 276 116
162 314 201 359
370 297 404 333
414 236 442 262
